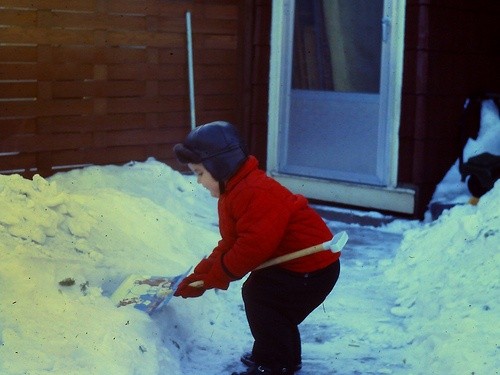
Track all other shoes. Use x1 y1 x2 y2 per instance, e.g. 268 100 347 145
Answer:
232 350 303 375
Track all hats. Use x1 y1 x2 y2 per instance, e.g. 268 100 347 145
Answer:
174 121 245 193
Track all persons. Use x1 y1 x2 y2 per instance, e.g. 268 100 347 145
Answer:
172 120 342 375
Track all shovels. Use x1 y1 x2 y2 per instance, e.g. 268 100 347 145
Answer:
108 229 348 318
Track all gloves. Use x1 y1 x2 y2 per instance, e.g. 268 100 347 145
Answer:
189 255 238 291
173 258 213 298
